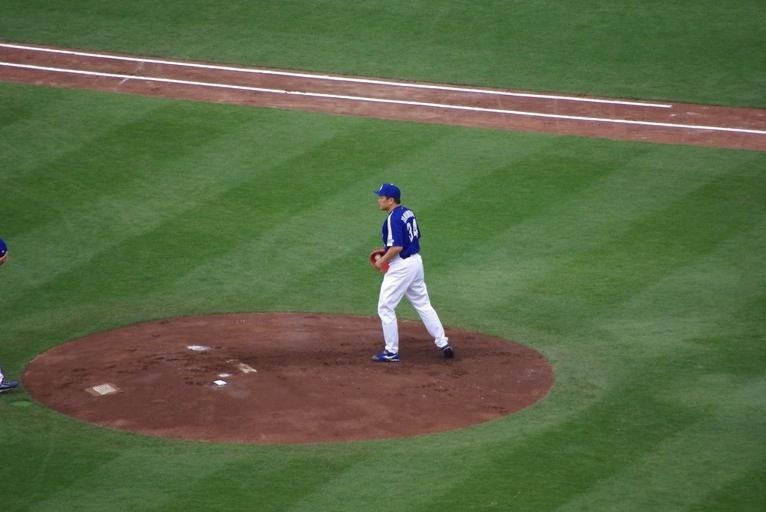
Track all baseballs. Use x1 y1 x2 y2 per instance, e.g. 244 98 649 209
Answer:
375 254 381 260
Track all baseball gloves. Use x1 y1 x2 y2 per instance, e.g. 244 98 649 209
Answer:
370 249 389 272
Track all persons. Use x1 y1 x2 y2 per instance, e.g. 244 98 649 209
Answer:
371 183 455 363
0 237 20 392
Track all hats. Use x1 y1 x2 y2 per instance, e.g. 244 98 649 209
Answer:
372 183 401 198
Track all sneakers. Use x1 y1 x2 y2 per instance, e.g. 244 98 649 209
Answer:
441 345 455 360
372 350 401 362
